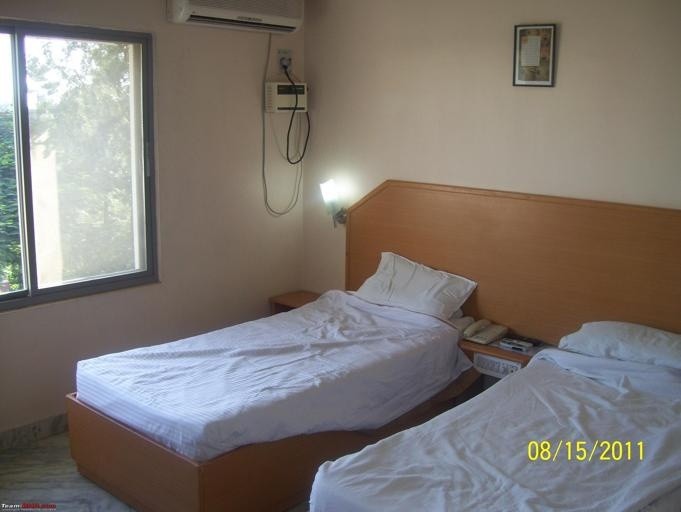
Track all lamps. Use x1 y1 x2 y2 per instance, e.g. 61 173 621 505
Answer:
321 178 346 228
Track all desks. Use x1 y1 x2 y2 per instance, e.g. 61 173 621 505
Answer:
460 339 531 384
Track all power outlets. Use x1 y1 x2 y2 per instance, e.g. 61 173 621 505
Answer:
278 49 293 74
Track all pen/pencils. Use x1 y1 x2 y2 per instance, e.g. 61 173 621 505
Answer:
512 347 523 351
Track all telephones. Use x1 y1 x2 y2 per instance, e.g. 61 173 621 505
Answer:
464 318 509 344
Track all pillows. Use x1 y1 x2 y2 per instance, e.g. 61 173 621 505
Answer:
354 251 478 319
559 321 681 370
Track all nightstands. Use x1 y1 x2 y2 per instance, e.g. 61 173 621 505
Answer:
269 291 320 314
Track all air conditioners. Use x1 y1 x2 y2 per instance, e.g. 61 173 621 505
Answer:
167 0 304 36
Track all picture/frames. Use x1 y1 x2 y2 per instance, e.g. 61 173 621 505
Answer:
513 23 556 88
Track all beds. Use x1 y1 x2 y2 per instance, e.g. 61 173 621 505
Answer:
65 289 481 512
309 349 681 512
